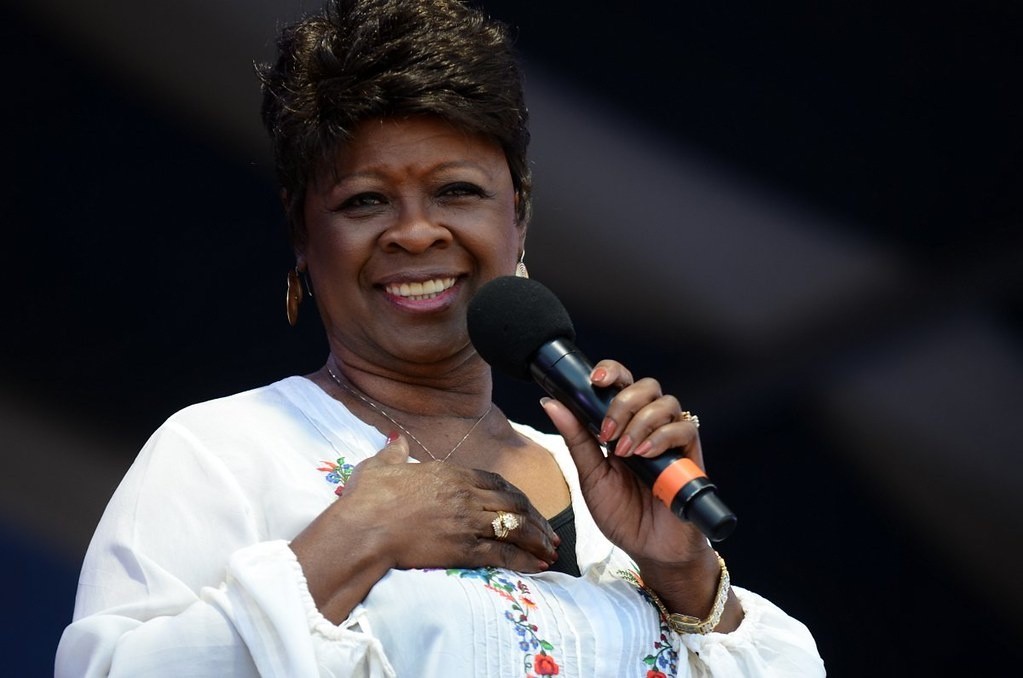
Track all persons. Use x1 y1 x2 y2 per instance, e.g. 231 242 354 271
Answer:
54 3 833 678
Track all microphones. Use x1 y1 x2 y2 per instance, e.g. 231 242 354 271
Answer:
465 274 739 543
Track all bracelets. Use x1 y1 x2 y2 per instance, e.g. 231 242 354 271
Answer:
645 550 732 634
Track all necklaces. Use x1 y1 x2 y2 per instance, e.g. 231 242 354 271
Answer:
321 357 491 458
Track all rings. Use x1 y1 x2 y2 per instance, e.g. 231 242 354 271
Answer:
489 510 518 540
680 410 701 430
